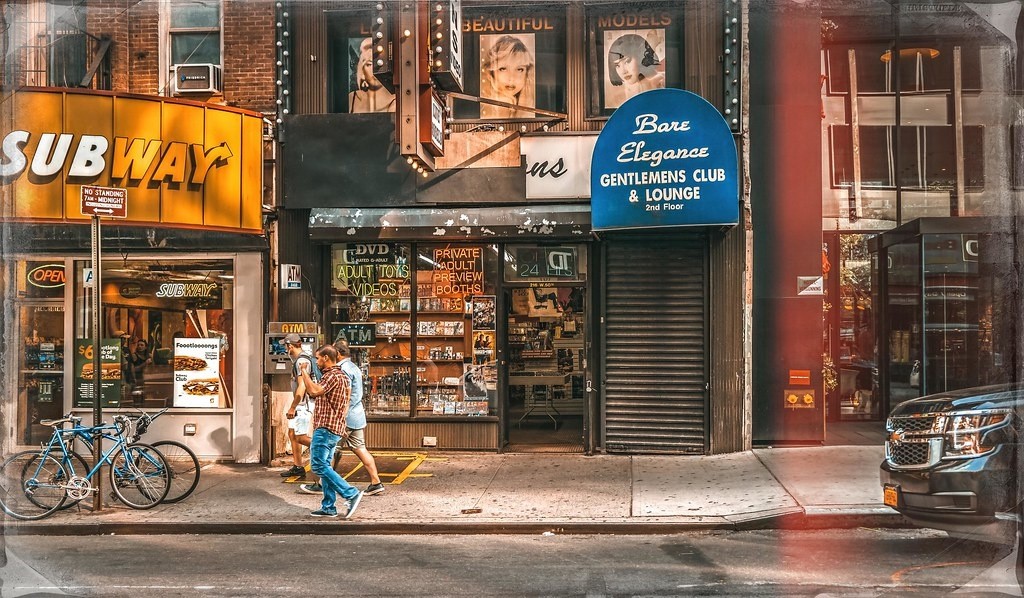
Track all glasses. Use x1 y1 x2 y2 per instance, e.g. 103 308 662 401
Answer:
119 333 126 338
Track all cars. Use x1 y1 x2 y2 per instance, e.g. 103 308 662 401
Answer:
838 356 874 399
880 381 1024 547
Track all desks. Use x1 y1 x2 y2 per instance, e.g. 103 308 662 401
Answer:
509 371 570 429
143 365 173 408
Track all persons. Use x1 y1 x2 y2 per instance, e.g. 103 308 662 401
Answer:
349 37 398 112
280 333 341 477
111 330 152 392
481 37 534 119
299 344 363 519
300 340 386 497
607 34 666 98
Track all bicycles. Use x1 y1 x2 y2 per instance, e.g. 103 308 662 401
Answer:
0 415 171 521
21 405 200 512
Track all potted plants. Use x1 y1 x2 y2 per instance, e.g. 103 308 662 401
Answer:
839 267 859 296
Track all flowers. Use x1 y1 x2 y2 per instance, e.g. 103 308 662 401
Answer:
821 18 838 50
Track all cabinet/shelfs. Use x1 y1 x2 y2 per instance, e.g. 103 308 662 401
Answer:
366 282 483 411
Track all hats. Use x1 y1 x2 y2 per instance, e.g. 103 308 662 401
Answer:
111 331 130 339
279 332 302 345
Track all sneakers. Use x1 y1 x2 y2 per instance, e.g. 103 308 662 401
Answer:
360 483 385 496
300 479 323 494
333 451 342 470
344 492 363 519
310 507 337 518
280 466 306 477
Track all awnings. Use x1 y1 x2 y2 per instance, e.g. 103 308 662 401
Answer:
593 86 741 227
307 205 592 241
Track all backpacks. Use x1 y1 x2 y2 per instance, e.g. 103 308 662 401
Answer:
292 355 322 414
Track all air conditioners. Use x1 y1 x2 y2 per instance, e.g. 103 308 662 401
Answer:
174 63 221 94
